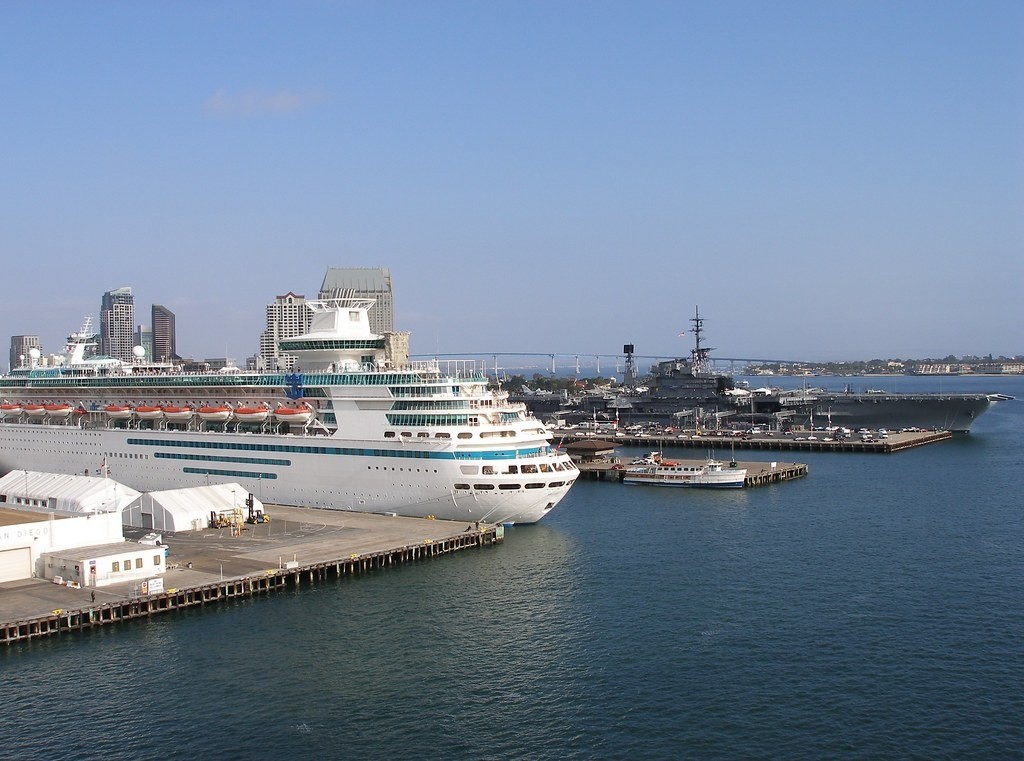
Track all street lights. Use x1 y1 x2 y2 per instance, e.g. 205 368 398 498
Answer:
231 489 237 513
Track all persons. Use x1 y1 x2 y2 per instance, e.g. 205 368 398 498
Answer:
91 589 95 602
844 384 847 396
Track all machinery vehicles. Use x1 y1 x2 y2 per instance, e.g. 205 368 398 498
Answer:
209 509 232 528
246 492 270 525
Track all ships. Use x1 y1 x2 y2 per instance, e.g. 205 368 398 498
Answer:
550 300 1019 433
0 283 583 524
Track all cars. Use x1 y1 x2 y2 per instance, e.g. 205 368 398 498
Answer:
575 423 888 441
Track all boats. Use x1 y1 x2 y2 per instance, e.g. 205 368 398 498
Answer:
195 407 230 421
43 403 71 416
620 457 747 489
106 404 130 418
23 403 47 416
163 405 192 420
135 406 163 419
0 404 24 415
272 405 313 423
231 408 269 422
